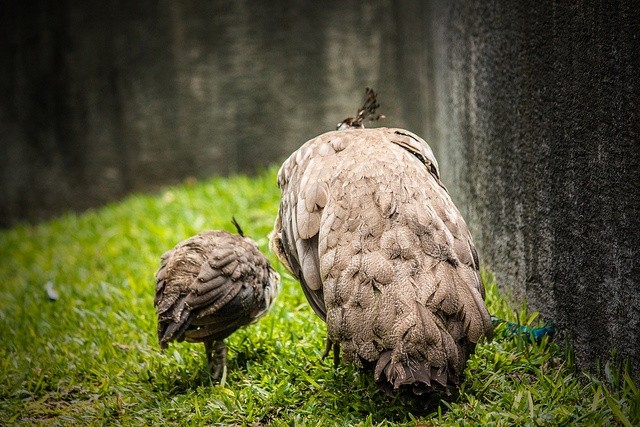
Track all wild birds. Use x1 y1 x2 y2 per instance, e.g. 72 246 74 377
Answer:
153 216 281 392
268 86 494 405
